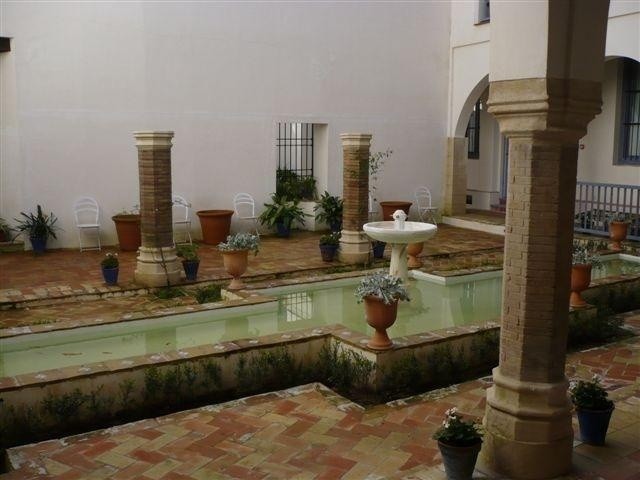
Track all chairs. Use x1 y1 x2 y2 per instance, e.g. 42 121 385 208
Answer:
74 196 103 252
369 146 394 222
415 186 439 225
172 195 192 245
233 193 259 233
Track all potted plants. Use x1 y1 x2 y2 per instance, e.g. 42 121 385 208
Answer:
313 190 344 232
606 211 636 251
570 250 603 307
111 201 143 251
316 232 339 263
11 204 67 259
215 232 261 291
178 245 201 280
370 240 386 261
100 253 123 285
433 407 486 480
257 192 313 235
0 218 25 255
354 268 413 350
571 375 616 446
